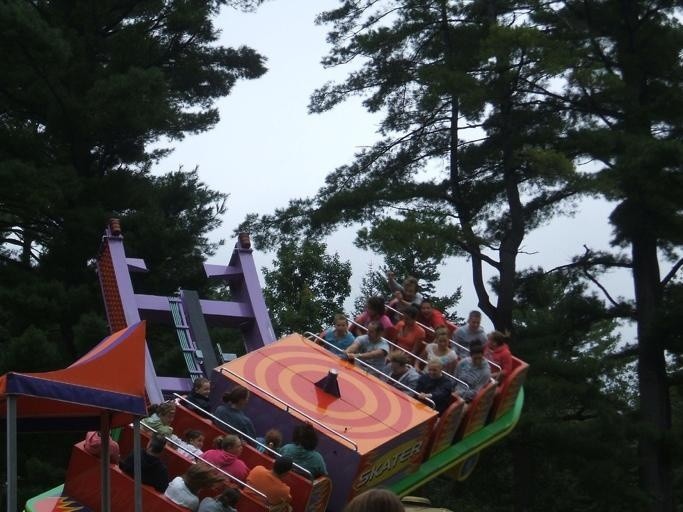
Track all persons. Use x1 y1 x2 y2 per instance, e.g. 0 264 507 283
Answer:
347 489 405 511
88 377 329 512
319 278 512 416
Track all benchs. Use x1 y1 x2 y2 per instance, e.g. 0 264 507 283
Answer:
63 429 195 511
389 293 528 420
168 387 335 511
316 324 464 459
346 299 503 436
117 418 291 511
